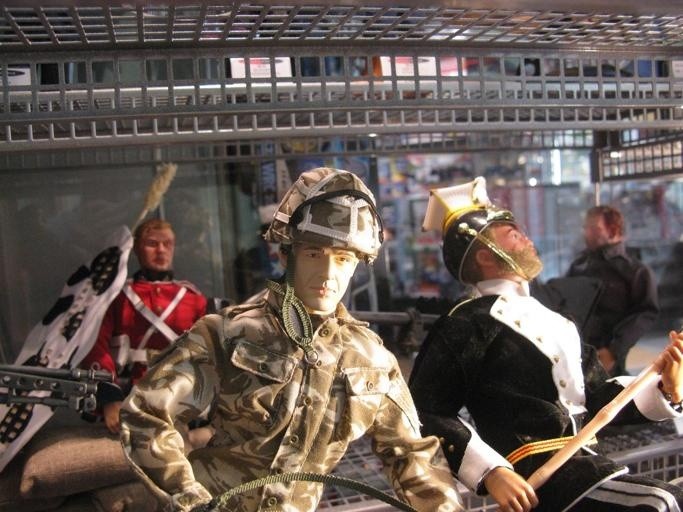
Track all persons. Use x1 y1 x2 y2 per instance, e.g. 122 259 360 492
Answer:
1 167 683 511
